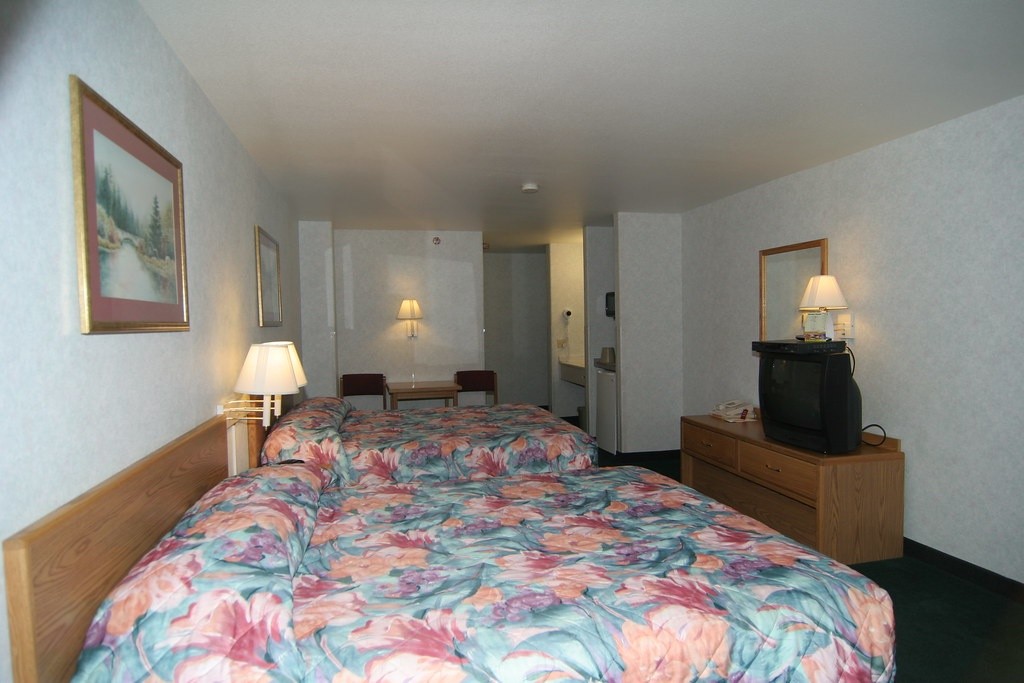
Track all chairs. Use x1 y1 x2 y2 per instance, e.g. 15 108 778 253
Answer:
454 369 499 407
340 374 387 408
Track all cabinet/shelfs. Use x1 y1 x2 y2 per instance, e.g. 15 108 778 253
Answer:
681 408 904 565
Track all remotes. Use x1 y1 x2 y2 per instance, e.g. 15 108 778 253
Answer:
796 335 832 341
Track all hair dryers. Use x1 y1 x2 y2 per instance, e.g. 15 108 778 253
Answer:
562 309 572 328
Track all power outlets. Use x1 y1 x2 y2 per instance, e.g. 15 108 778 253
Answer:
839 321 855 339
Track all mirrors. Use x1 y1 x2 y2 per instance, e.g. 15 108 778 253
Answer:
758 238 828 341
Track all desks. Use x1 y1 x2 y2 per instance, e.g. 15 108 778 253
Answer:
387 379 461 409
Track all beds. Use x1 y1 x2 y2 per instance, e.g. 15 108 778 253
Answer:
0 413 900 683
242 392 598 488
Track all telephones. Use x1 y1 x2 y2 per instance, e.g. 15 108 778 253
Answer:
716 400 754 417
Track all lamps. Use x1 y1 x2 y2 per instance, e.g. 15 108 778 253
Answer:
798 275 848 340
397 298 424 338
228 340 308 418
217 344 300 431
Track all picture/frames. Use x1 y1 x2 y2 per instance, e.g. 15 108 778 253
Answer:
70 75 191 335
254 224 283 327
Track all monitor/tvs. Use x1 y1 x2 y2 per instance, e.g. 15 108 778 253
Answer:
758 353 863 456
